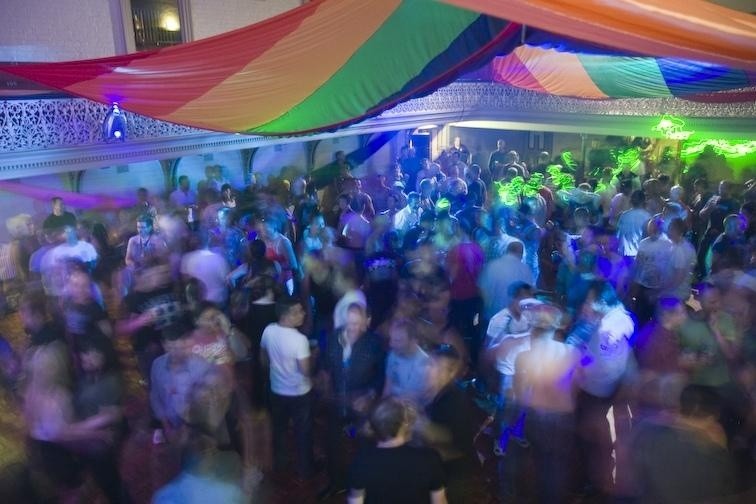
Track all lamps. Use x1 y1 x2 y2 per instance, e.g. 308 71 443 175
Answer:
104 103 128 141
